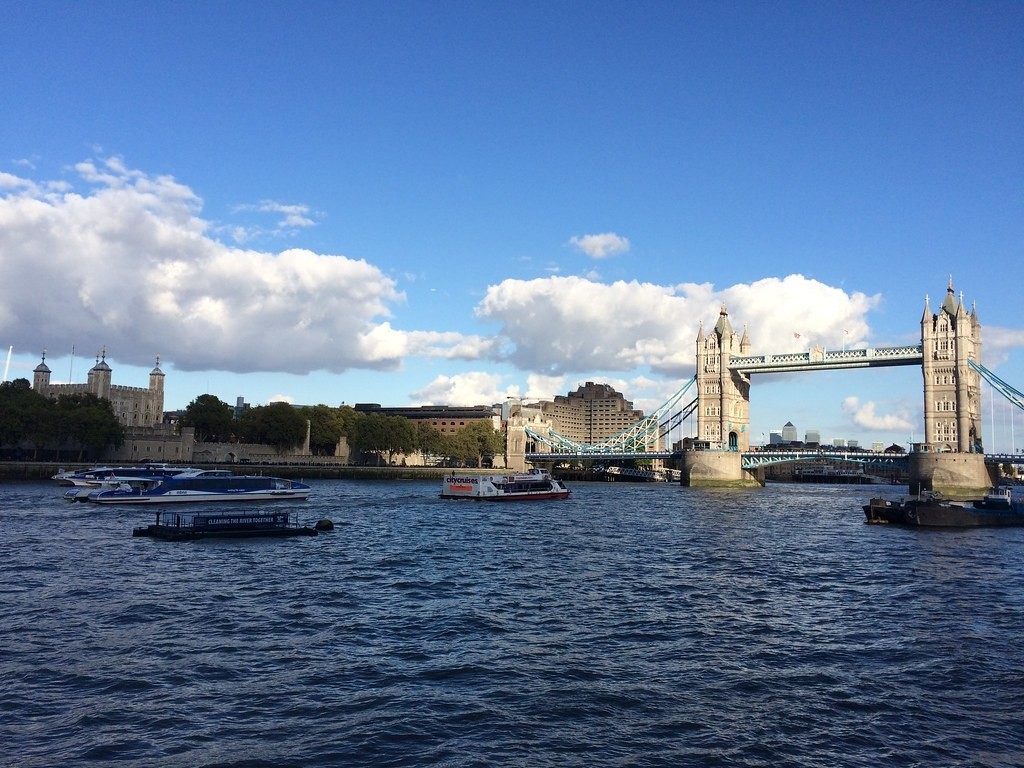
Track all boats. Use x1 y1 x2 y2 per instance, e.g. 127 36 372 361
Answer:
438 474 572 501
62 470 312 504
513 468 553 480
51 463 202 487
862 487 1024 529
